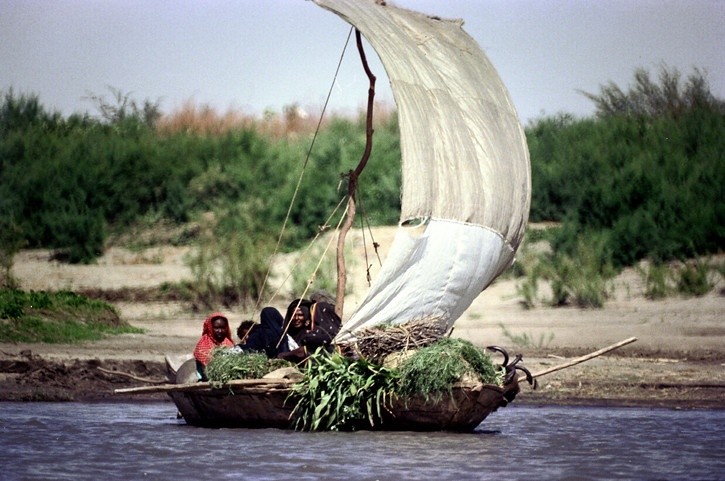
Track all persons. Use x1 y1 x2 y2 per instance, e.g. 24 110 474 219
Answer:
278 334 322 374
311 292 335 314
234 320 256 353
284 299 315 345
247 307 289 359
194 313 235 382
298 303 341 362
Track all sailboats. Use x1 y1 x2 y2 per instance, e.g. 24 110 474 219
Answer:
160 0 539 434
